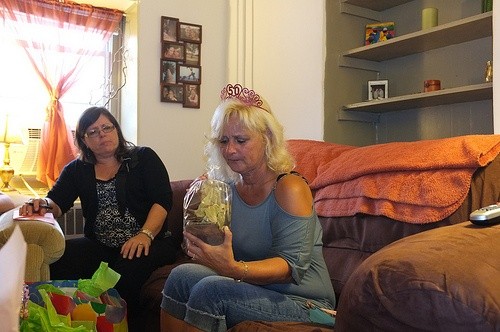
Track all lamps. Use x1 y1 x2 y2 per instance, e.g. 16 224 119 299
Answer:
0 110 25 192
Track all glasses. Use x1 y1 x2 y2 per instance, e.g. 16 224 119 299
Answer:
83 125 116 138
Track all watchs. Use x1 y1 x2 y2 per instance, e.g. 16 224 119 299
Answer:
138 229 154 240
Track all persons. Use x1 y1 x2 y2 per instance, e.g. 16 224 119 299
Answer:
0 192 16 215
160 84 337 332
190 29 198 40
188 90 198 104
19 107 173 332
374 88 384 98
163 88 174 100
166 46 181 58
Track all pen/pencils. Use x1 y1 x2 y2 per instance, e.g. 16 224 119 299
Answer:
24 201 52 208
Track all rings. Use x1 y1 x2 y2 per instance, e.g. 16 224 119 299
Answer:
193 254 196 258
139 243 143 245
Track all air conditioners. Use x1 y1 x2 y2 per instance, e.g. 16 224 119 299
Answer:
22 123 76 179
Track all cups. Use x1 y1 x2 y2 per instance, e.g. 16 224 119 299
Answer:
424 79 440 92
481 0 493 13
421 7 438 30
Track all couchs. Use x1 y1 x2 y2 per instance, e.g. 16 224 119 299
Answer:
139 137 500 332
0 192 64 283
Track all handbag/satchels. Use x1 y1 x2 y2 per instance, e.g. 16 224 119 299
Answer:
20 261 128 332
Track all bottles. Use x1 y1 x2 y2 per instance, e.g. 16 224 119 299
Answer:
485 60 493 83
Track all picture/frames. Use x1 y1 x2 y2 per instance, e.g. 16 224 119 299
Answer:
368 80 389 100
160 16 202 109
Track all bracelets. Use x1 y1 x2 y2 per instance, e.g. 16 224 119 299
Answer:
239 260 248 281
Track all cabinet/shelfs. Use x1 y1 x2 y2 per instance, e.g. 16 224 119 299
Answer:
342 12 493 112
